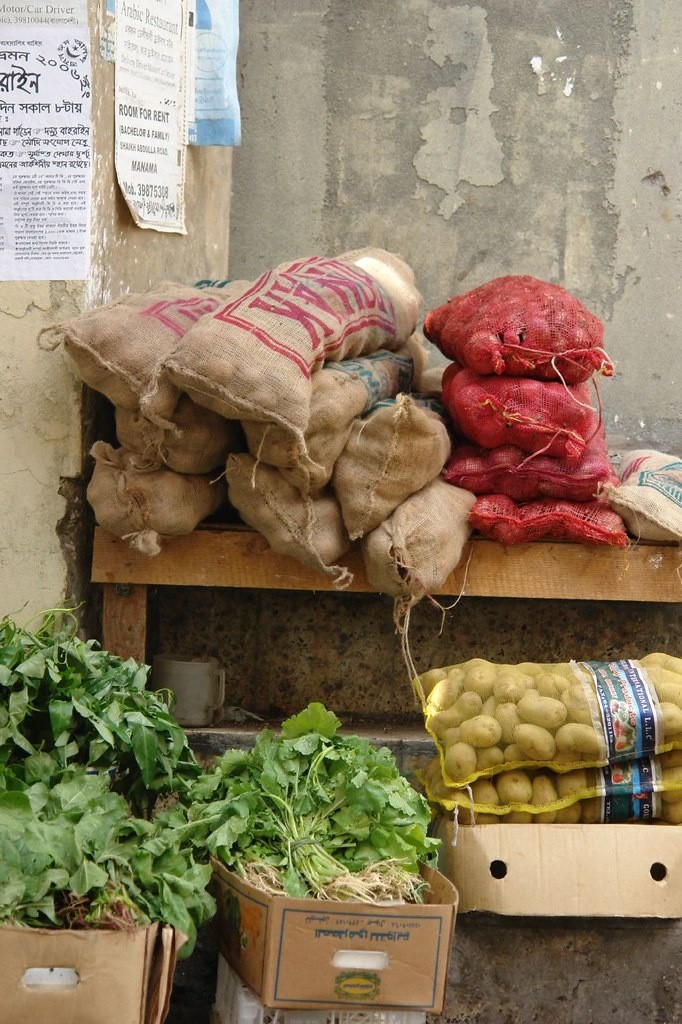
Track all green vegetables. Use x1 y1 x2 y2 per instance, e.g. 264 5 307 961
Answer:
0 601 441 954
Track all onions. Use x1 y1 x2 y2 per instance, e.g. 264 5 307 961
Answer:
420 274 625 538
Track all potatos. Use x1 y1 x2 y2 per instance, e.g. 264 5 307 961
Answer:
414 652 682 828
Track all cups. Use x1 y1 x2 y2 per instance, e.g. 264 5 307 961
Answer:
150 653 226 727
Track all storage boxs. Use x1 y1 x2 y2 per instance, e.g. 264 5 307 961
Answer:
430 814 682 926
0 910 191 1024
197 856 459 1015
